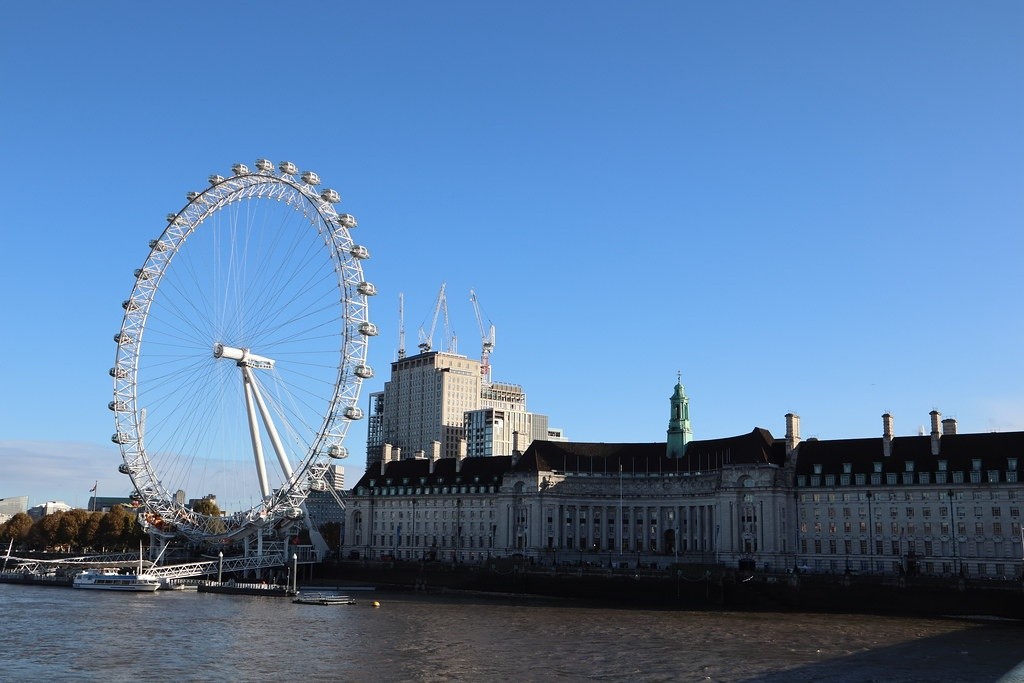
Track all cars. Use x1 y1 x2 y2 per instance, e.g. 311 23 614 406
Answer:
842 567 860 576
784 565 811 574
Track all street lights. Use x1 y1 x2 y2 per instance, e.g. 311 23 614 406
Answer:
608 547 613 568
579 545 584 563
637 548 641 568
553 548 557 566
792 550 800 573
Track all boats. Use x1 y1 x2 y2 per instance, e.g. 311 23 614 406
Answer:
197 551 300 597
292 592 357 605
72 568 161 592
